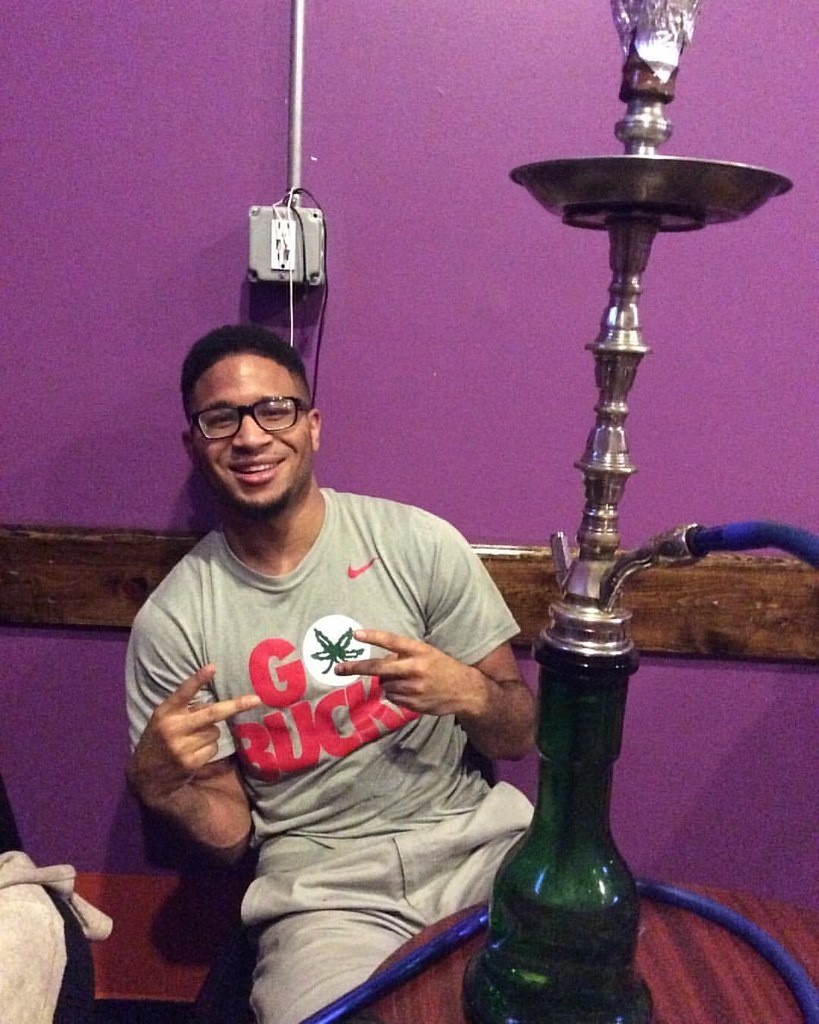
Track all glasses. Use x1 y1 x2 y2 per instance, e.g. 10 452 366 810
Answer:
188 397 310 441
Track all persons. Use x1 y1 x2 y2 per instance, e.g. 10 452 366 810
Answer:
127 324 535 1024
0 779 115 1024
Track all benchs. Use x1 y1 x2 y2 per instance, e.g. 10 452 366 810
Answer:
0 873 239 1002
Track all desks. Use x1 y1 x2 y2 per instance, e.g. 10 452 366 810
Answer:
350 881 819 1024
